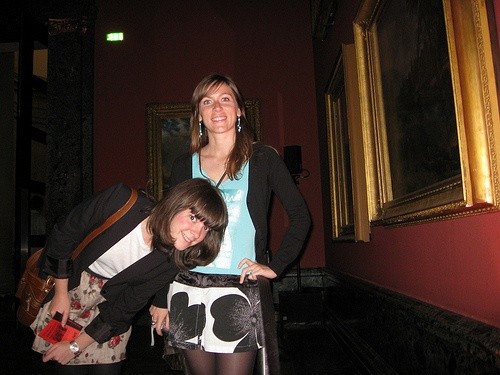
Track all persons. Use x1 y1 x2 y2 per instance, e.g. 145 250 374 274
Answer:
27 178 229 375
148 73 313 371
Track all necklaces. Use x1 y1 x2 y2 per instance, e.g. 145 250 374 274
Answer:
199 148 228 189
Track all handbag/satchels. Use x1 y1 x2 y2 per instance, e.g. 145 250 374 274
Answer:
17 247 57 325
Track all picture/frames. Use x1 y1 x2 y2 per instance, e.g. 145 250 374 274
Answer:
144 99 262 202
350 0 500 228
322 41 371 243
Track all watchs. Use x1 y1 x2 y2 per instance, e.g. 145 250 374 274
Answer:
69 339 82 356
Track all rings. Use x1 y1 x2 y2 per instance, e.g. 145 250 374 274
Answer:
152 320 157 325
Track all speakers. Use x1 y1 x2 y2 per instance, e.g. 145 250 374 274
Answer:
283 145 303 175
